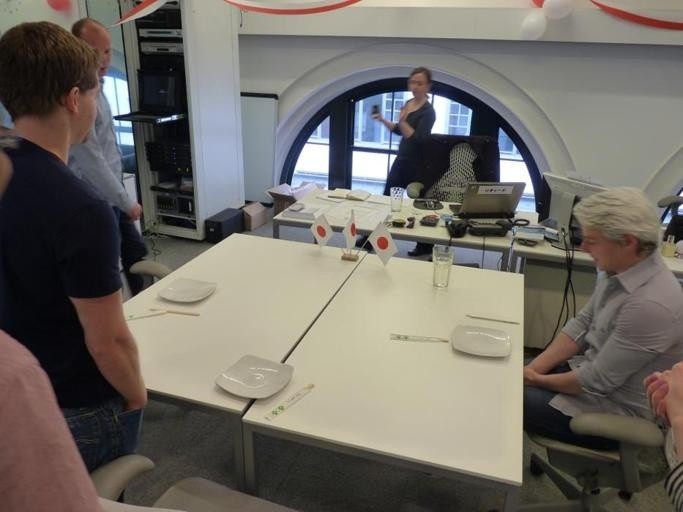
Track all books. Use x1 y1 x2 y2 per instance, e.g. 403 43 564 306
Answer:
328 188 371 201
284 203 328 219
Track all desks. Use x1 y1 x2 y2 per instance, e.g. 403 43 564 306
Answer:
274 189 539 272
121 234 366 494
242 251 523 511
515 229 682 349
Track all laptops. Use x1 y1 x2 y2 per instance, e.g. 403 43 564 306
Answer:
449 182 526 219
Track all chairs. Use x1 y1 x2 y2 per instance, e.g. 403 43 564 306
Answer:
406 133 499 203
525 412 672 511
90 455 296 512
128 261 172 291
659 195 682 240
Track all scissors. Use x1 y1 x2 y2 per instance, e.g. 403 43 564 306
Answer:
515 239 538 247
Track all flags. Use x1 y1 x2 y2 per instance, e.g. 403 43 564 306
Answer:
357 222 398 266
342 208 357 256
310 214 346 256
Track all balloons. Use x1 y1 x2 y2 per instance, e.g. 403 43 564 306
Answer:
543 0 574 19
520 8 545 42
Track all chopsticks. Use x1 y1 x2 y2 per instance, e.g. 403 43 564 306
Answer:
264 382 314 420
466 314 520 326
125 310 166 321
390 333 448 344
150 308 200 316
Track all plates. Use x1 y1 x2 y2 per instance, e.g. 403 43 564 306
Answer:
215 353 295 400
450 325 511 358
159 276 217 304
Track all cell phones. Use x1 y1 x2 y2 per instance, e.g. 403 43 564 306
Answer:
371 105 380 117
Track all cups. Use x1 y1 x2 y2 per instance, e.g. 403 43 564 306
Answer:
390 187 405 212
432 246 454 288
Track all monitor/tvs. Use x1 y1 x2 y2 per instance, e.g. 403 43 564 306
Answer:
538 173 609 251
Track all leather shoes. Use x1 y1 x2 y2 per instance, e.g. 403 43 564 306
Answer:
356 235 373 251
408 241 434 256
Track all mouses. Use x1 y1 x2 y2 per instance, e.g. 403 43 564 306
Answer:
425 200 437 209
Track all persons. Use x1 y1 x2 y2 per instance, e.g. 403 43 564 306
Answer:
524 186 683 454
0 21 147 505
643 362 683 512
355 67 436 256
68 19 154 298
0 330 101 511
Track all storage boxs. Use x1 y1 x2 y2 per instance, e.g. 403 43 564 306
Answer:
241 201 268 231
267 182 325 215
205 209 245 243
